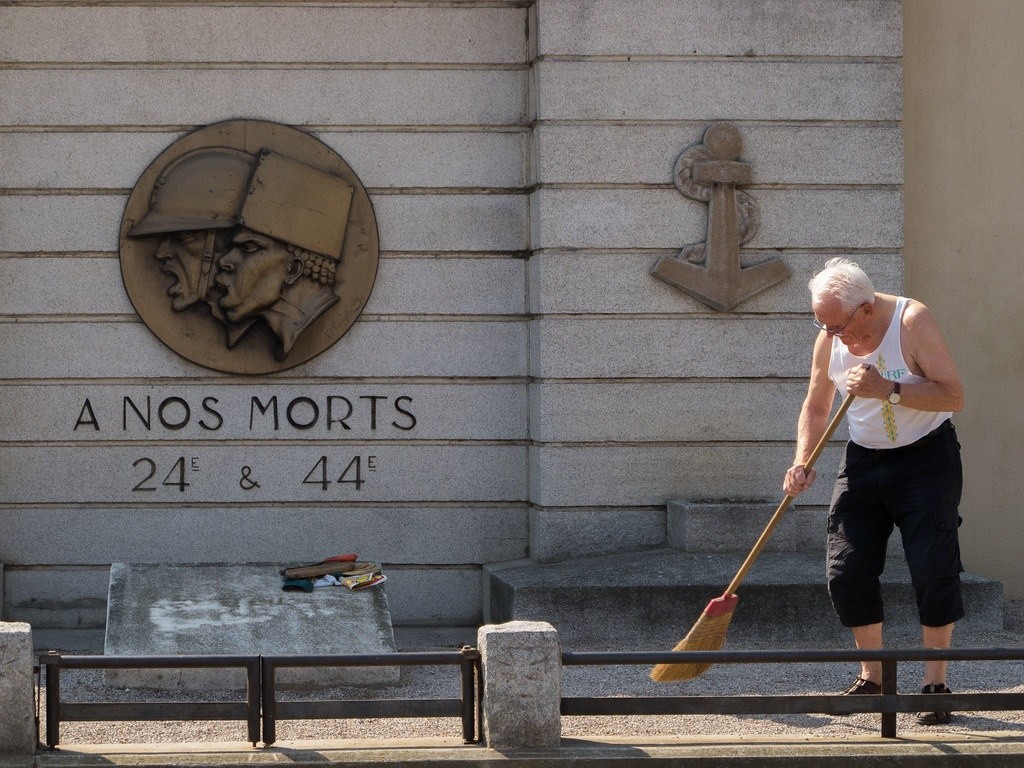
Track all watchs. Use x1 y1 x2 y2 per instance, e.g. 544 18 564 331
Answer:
888 381 902 406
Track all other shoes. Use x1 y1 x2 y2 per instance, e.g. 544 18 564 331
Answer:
837 676 876 696
915 684 951 723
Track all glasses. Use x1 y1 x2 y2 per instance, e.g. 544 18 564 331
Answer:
812 304 865 337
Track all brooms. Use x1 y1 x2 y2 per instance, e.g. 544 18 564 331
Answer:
649 363 872 681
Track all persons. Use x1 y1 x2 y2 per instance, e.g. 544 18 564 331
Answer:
783 254 965 726
214 149 354 363
149 146 264 350
